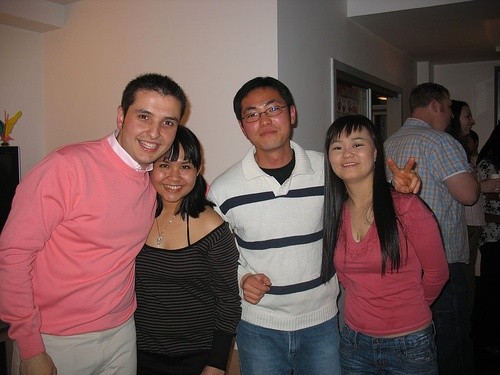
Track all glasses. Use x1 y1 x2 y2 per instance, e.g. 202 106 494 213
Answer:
240 103 289 123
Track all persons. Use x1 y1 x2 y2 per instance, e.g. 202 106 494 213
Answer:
382 82 500 375
207 76 423 375
318 115 448 375
133 124 241 375
0 72 186 374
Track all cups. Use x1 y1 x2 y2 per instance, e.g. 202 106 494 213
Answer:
489 174 500 191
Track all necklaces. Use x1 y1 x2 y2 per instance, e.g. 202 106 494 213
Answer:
155 213 177 246
